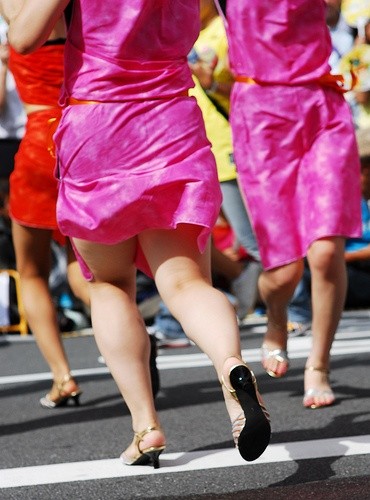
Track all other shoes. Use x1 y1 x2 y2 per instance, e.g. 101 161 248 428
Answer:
286 319 310 338
146 325 190 348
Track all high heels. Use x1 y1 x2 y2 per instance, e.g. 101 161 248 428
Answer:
39 375 82 409
302 358 336 408
259 308 290 378
119 424 168 469
221 355 272 463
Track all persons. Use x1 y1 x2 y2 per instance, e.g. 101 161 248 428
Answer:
8 0 273 468
0 0 160 406
199 0 362 408
0 0 370 369
